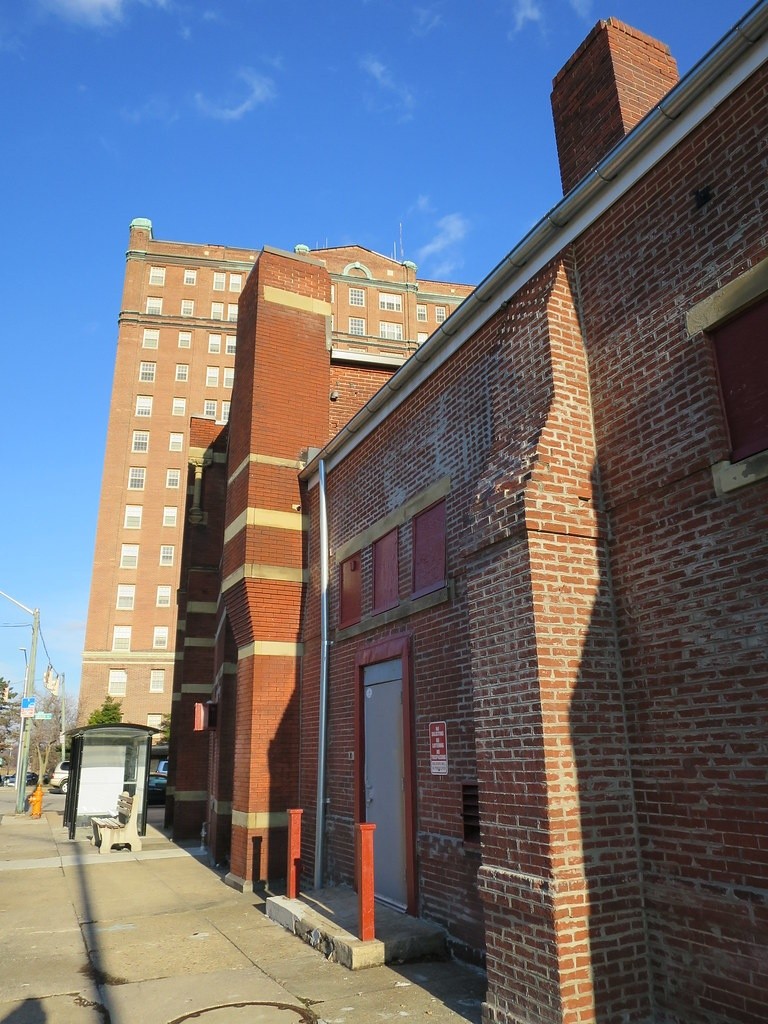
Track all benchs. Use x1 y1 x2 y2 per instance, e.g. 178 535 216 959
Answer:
90 791 142 854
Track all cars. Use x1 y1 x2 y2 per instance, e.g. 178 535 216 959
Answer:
0 772 50 788
147 772 168 805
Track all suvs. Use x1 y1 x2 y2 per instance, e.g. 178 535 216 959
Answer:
155 757 168 774
49 760 70 793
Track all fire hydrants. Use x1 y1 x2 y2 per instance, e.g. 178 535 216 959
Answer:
27 784 43 818
